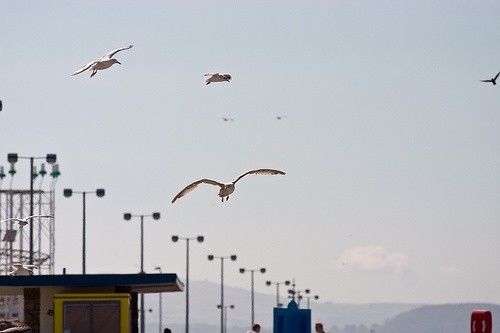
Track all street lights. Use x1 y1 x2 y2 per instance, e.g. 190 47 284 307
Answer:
123 212 161 333
207 254 237 333
218 304 235 333
172 235 204 333
63 188 105 274
266 280 290 308
7 152 57 275
288 289 319 309
239 267 266 328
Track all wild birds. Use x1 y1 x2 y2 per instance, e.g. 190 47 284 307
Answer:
204 73 231 85
72 45 134 77
171 169 286 205
480 71 500 85
